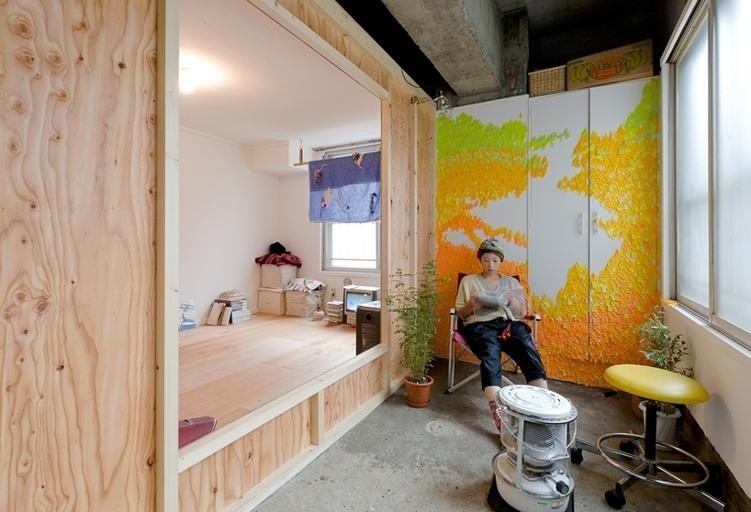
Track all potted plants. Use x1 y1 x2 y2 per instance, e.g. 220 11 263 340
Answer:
384 258 444 408
638 307 693 448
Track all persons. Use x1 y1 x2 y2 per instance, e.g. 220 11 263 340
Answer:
455 236 550 434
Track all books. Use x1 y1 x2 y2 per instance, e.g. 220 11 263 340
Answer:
327 301 344 326
473 294 510 309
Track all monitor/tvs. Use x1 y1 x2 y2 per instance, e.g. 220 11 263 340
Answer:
344 284 382 326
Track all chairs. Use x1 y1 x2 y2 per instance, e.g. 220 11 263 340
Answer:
444 272 541 394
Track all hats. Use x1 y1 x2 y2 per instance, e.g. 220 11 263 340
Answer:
479 237 504 255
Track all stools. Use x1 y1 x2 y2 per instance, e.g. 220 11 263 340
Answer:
570 364 730 512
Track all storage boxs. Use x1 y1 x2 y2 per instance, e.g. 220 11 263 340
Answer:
567 39 653 90
257 262 322 318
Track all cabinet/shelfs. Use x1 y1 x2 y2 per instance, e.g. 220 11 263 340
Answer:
529 75 662 395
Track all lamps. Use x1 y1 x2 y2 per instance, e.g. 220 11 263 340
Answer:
411 93 451 111
